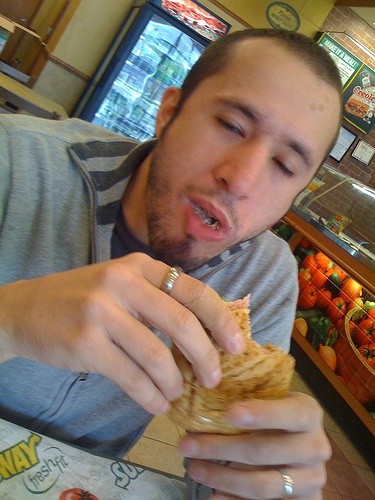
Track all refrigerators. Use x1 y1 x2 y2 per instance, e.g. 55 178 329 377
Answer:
70 0 232 144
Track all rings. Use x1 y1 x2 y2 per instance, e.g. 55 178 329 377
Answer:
160 265 183 292
278 468 293 494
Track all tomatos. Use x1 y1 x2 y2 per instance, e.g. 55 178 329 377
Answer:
298 253 375 372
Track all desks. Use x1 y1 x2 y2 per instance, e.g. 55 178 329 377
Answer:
0 72 68 120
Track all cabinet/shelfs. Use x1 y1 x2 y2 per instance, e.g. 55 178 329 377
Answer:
269 163 375 474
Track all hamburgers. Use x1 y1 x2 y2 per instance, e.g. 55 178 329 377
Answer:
165 294 296 436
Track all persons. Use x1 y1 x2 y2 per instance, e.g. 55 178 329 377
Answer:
1 28 344 500
